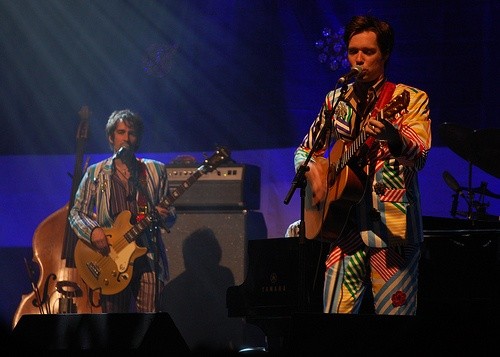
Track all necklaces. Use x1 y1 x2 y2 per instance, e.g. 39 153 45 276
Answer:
118 162 130 175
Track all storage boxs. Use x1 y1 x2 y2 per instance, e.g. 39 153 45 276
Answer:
161 211 267 351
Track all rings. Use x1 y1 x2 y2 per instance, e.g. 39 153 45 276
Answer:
369 125 375 131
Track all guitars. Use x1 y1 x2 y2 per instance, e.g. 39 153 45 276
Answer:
73 140 229 297
302 90 410 237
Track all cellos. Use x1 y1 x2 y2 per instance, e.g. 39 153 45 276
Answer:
11 105 106 328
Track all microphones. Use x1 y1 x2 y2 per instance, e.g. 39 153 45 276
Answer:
442 171 466 198
338 66 363 83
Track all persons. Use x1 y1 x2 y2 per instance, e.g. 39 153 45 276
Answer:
293 18 432 315
69 108 178 313
285 219 303 238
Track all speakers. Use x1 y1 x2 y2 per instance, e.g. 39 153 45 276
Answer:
152 209 261 357
2 312 192 357
